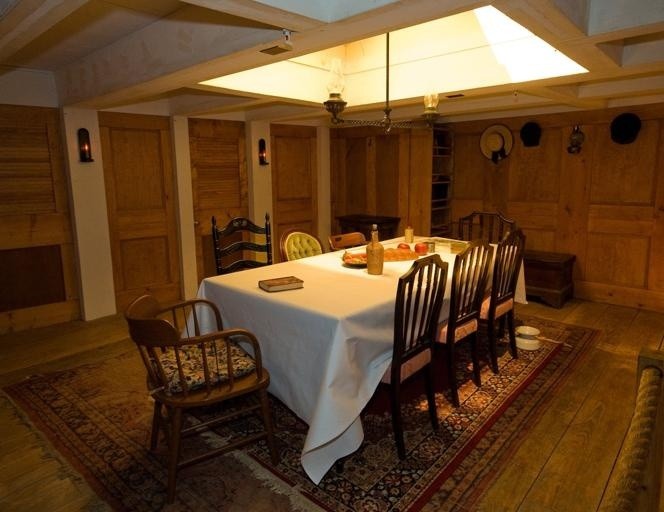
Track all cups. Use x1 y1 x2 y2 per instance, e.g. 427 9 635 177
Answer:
428 242 435 253
405 229 414 243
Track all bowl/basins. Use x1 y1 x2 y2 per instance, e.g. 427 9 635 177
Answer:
515 337 541 351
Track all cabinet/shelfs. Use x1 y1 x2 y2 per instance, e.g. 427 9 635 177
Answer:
408 127 452 237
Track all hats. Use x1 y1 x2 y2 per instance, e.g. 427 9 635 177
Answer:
479 124 513 164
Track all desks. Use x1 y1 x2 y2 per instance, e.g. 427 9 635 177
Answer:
524 246 576 310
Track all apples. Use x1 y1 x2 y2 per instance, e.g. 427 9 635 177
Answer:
415 243 427 255
398 244 409 250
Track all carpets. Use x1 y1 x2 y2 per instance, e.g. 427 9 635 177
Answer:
0 311 600 510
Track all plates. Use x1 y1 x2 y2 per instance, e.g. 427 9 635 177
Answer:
515 326 541 338
343 256 367 267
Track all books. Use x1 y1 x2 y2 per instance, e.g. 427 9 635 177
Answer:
257 275 304 292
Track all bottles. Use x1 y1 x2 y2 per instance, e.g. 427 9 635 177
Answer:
366 224 384 275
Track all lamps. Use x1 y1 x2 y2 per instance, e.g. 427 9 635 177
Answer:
257 138 269 165
323 33 440 133
76 126 95 163
